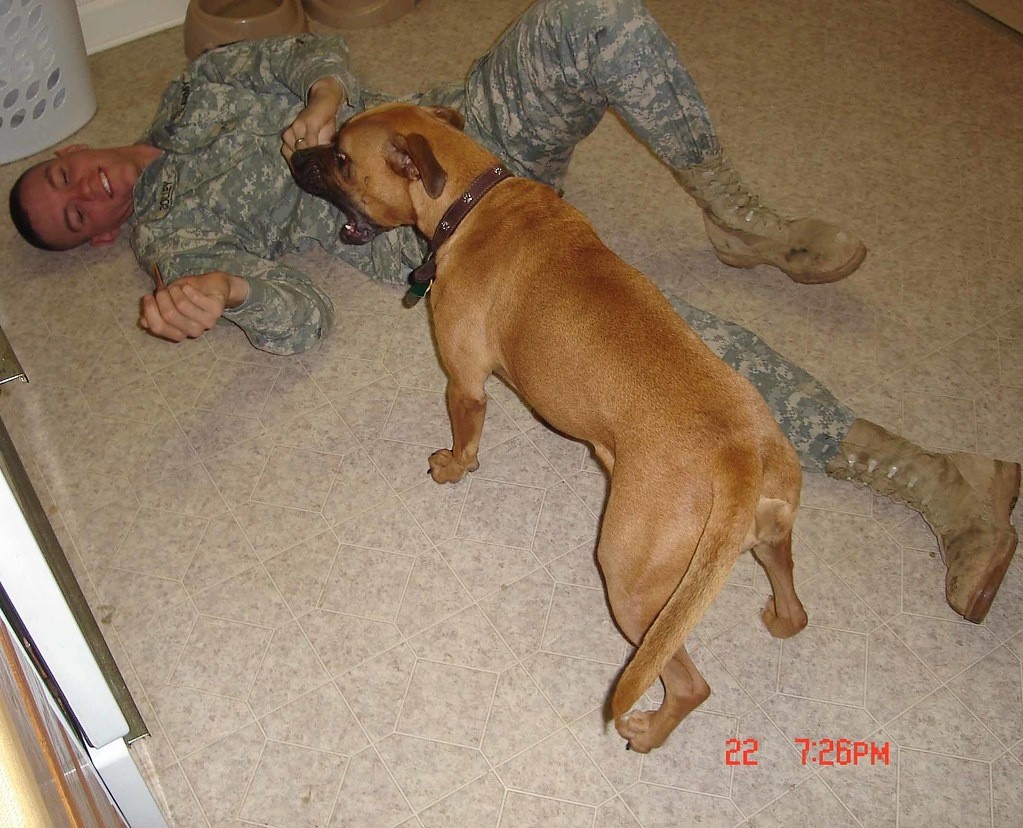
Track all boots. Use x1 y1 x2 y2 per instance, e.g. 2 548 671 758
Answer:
825 418 1021 624
672 151 866 285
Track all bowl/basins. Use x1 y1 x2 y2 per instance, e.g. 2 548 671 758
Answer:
302 0 416 28
184 0 308 61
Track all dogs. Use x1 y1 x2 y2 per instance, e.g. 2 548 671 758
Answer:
290 102 811 756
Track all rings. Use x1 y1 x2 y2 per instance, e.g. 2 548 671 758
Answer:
295 138 305 148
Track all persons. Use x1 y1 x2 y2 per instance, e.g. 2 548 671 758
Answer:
8 0 1023 624
